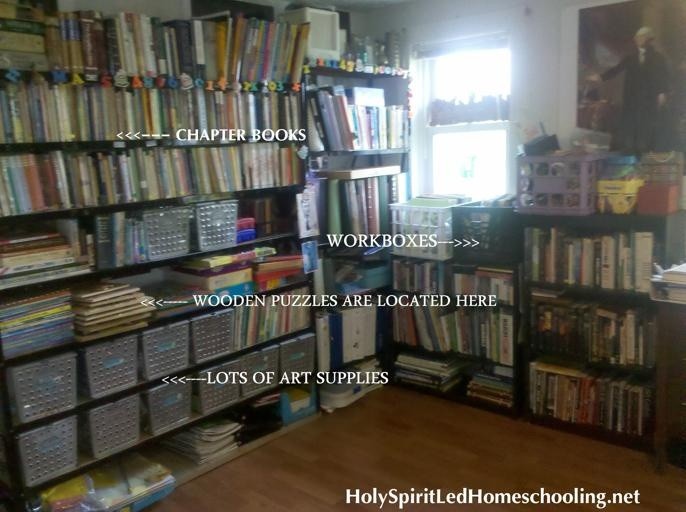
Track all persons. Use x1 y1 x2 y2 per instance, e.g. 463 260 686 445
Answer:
296 191 321 239
302 241 319 274
593 27 676 147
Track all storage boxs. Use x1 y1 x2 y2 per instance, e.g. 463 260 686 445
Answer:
139 373 193 437
189 307 238 364
141 203 193 261
189 200 239 252
239 344 280 397
13 413 79 488
79 391 141 460
2 349 80 426
138 317 192 381
279 331 316 382
388 194 480 262
81 333 139 400
450 200 525 265
515 151 610 214
194 354 242 415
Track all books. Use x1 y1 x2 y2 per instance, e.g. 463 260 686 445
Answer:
461 194 520 252
356 360 383 394
650 259 686 304
0 81 300 143
141 247 304 319
316 301 376 373
0 211 146 289
391 258 515 367
303 85 408 151
640 151 683 185
0 143 304 216
314 166 406 242
404 192 471 254
529 354 657 435
528 286 658 368
233 288 311 351
0 281 156 361
315 247 391 298
0 0 311 84
525 226 661 295
393 351 513 410
26 451 174 512
253 385 311 419
166 421 242 465
236 194 293 245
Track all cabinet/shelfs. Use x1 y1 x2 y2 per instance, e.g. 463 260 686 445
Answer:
0 9 323 511
518 206 686 457
389 234 523 420
305 56 411 383
